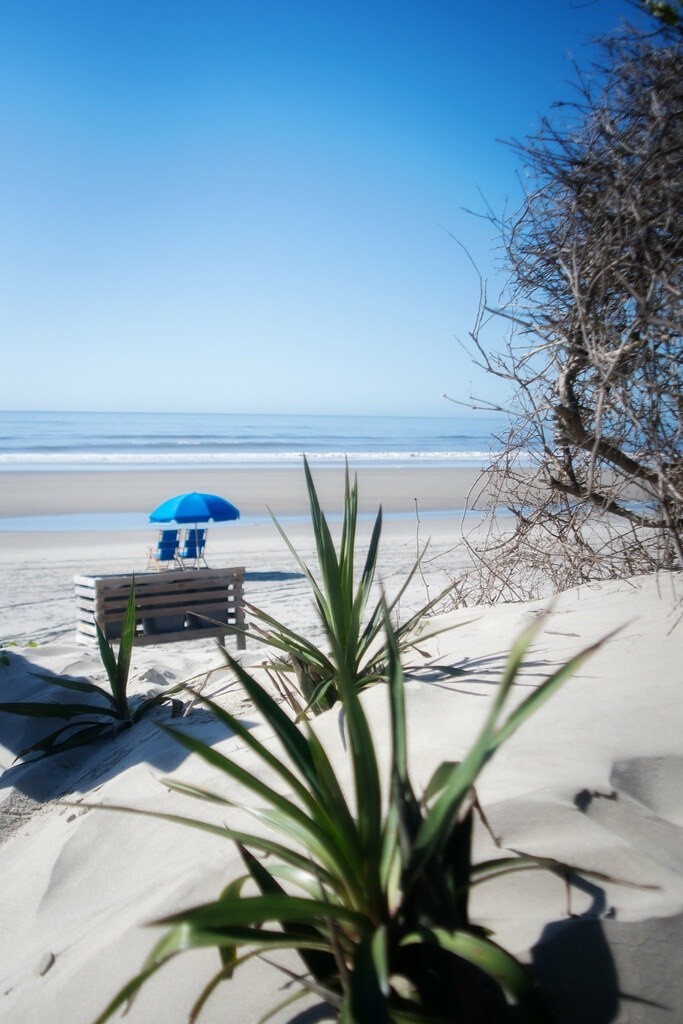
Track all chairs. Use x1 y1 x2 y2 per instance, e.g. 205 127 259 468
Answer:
173 528 209 570
146 528 183 572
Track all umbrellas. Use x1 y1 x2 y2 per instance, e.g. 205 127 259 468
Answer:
148 490 239 570
